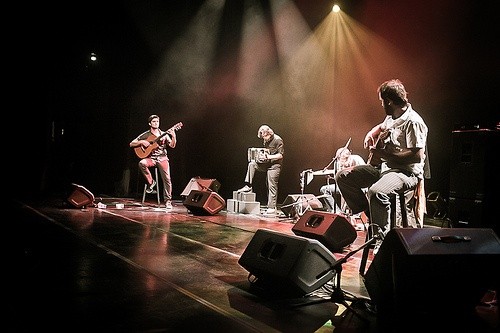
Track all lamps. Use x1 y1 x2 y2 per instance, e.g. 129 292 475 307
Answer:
91 53 97 61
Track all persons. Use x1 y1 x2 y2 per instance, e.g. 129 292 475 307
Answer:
130 115 176 208
320 148 368 214
335 79 428 256
237 125 284 210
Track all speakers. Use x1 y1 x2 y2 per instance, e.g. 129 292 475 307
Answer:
291 211 358 250
66 183 94 208
447 130 500 231
238 228 340 294
364 227 500 333
281 195 322 217
182 189 226 215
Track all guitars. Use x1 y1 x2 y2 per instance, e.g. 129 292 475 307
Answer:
134 121 183 159
366 127 390 166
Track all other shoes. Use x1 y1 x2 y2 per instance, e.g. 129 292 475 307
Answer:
373 227 390 255
165 201 172 208
146 180 156 193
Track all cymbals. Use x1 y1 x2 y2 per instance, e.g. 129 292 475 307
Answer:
312 168 334 176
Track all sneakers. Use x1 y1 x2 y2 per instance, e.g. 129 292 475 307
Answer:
266 206 277 214
238 185 252 193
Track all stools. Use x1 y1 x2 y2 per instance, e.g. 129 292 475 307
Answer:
142 166 160 204
359 179 420 274
